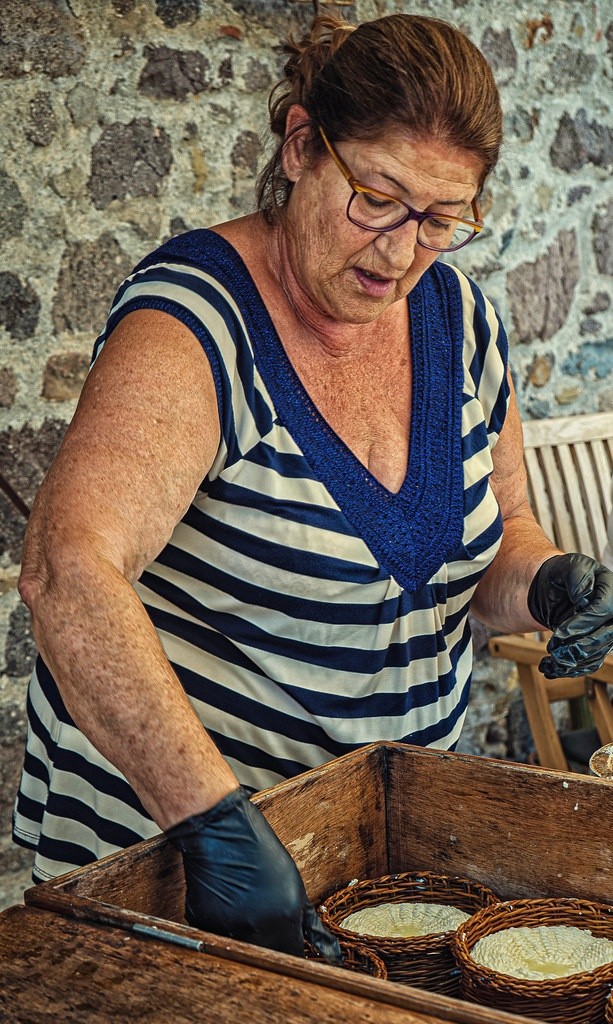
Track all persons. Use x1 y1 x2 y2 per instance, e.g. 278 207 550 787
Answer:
10 16 613 968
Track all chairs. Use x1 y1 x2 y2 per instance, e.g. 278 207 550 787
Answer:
486 410 613 772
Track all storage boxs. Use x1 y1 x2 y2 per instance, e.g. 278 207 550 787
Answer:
0 740 612 1022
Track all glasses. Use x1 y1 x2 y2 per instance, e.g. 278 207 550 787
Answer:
314 118 484 253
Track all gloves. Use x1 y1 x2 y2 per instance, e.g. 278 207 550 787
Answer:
163 788 344 960
527 552 613 679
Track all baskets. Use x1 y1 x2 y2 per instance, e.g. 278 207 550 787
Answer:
295 938 387 984
450 896 613 1024
316 870 502 998
